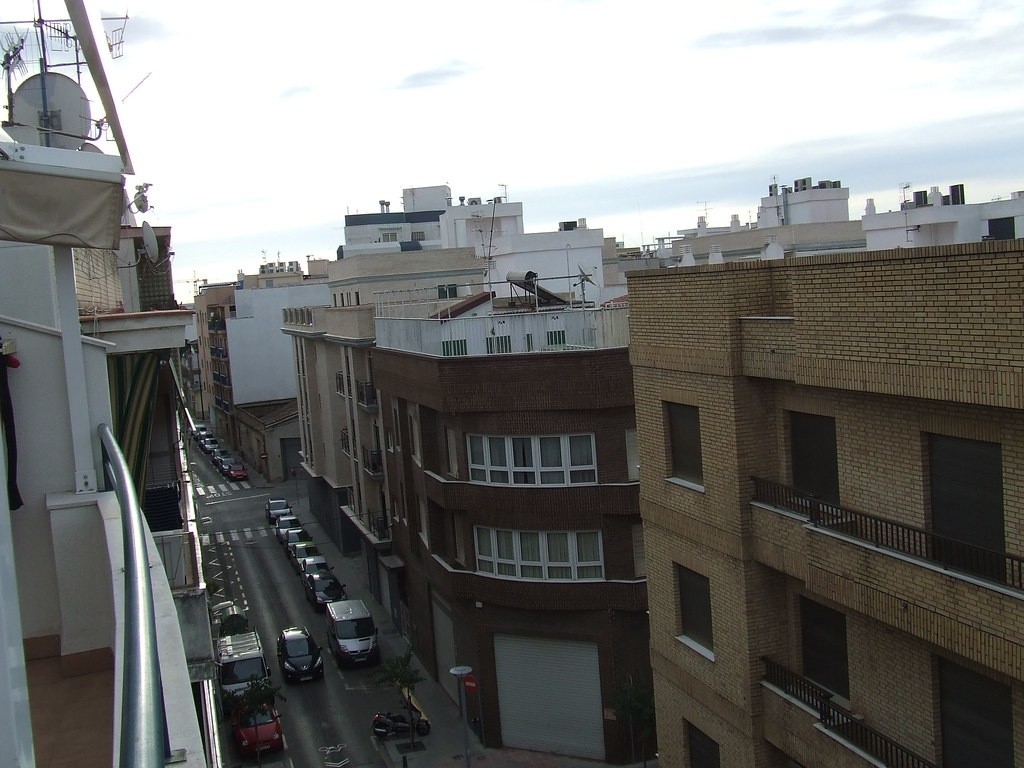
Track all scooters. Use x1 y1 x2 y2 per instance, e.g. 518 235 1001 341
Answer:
371 701 429 739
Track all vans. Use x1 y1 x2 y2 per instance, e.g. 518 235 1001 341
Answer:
325 598 379 663
216 628 272 713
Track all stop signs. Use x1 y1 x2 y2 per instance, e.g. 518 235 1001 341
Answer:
463 676 477 692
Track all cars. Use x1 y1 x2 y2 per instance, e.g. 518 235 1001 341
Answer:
228 700 284 758
265 496 347 613
190 423 247 481
275 625 325 680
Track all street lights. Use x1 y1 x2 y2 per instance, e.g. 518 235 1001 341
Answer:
451 666 473 768
